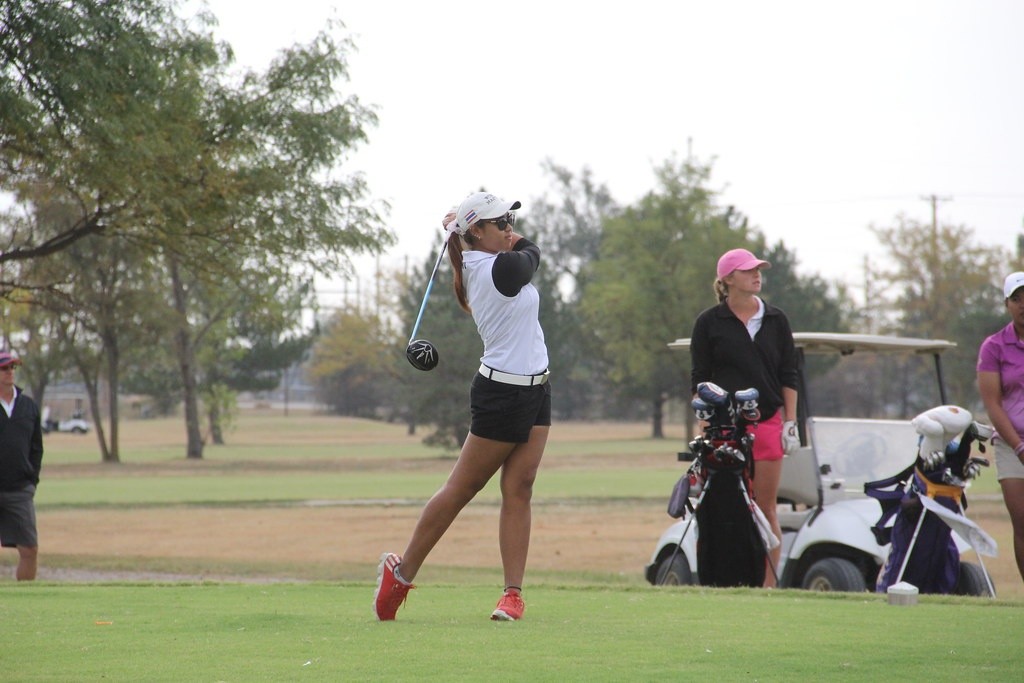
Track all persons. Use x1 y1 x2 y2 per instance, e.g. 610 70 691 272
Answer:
688 249 798 588
977 272 1024 583
0 350 42 580
372 191 551 620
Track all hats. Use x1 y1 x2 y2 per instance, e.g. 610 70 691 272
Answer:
1004 272 1024 300
717 248 769 282
457 192 521 231
0 352 17 366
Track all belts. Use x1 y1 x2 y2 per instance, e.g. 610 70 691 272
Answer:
478 363 551 386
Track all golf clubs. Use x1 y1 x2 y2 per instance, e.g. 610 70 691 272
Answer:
687 380 761 470
911 404 997 486
404 229 455 373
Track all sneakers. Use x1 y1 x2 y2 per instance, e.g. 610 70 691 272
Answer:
373 553 418 620
490 589 524 621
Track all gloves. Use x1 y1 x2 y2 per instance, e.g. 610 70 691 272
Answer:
442 210 465 237
781 421 801 455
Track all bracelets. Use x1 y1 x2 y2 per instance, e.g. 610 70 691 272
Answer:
1015 442 1024 454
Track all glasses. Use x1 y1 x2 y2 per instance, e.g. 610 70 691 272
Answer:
479 213 515 231
0 363 17 370
